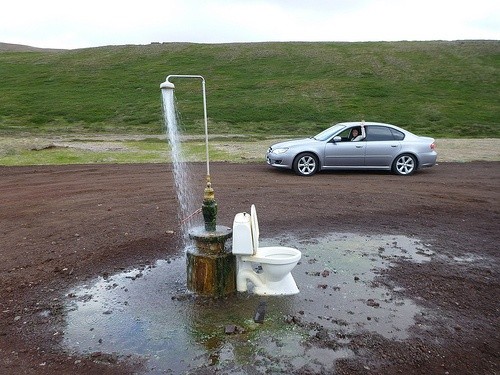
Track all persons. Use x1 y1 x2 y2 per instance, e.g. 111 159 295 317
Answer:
349 120 367 141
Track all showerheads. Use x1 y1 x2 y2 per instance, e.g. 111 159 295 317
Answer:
160 80 175 89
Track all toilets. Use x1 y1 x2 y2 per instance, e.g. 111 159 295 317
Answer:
232 204 302 295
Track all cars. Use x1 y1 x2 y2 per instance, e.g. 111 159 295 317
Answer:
267 122 437 179
